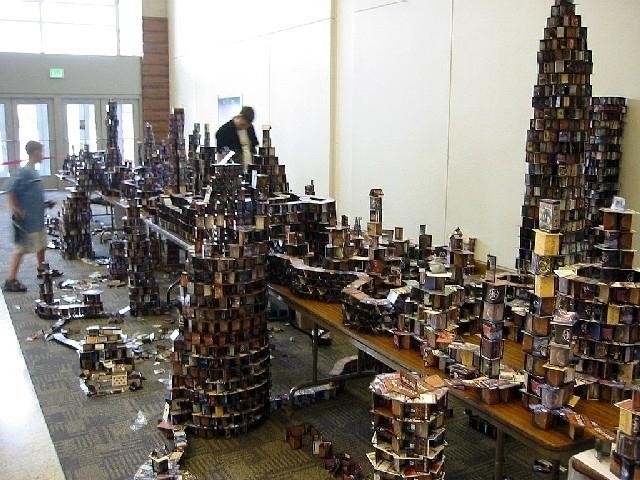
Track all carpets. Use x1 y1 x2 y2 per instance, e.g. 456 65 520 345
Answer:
0 190 570 479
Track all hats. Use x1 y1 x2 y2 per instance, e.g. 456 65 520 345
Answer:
25 141 41 152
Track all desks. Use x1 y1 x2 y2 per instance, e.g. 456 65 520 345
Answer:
62 176 619 480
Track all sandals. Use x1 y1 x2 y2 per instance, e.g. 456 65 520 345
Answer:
37 268 63 278
4 280 27 292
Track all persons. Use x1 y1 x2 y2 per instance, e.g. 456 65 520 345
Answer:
214 104 261 175
2 141 63 292
